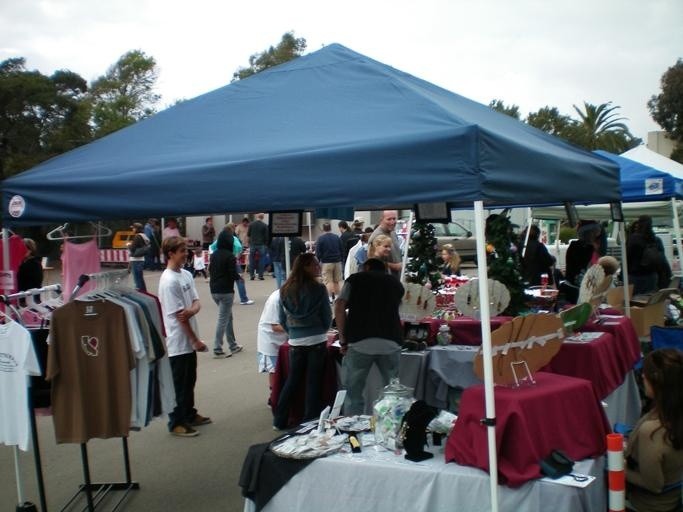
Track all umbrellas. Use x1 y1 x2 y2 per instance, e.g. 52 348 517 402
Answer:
400 150 683 283
591 143 682 297
0 42 630 512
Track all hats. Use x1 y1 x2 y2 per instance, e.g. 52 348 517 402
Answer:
129 223 144 228
24 237 37 251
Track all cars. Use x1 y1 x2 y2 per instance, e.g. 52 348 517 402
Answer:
395 218 477 268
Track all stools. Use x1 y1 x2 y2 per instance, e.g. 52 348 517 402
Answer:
584 313 641 367
444 369 612 487
549 329 629 396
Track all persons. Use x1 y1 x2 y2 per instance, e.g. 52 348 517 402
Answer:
257 288 288 409
248 213 270 281
334 259 406 417
189 249 211 283
209 232 243 360
590 237 603 264
630 221 640 241
352 220 364 242
626 215 659 296
232 217 250 272
315 223 342 299
338 221 354 281
161 217 181 267
655 235 674 290
565 224 601 286
211 226 255 305
16 237 43 308
441 243 461 276
366 226 374 233
518 224 556 286
201 217 216 249
271 236 288 288
272 252 334 432
622 347 683 511
368 210 403 282
143 218 157 271
355 234 369 273
368 234 403 275
290 229 307 271
128 223 150 292
158 235 212 438
151 220 164 270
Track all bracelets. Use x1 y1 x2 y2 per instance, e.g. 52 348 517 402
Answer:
338 342 349 346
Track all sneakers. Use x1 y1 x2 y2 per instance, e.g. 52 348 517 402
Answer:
259 276 264 280
183 414 211 426
240 300 255 305
169 424 200 436
250 275 255 280
213 351 232 359
230 344 247 353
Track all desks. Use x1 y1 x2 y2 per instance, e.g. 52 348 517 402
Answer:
237 415 607 512
331 340 428 405
425 343 485 411
425 312 512 346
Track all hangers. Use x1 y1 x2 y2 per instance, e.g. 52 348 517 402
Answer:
0 230 15 236
0 285 59 512
46 221 112 240
53 268 139 512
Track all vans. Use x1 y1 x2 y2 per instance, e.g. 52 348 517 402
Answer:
112 230 138 249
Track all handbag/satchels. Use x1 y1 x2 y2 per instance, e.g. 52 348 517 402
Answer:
135 247 145 256
